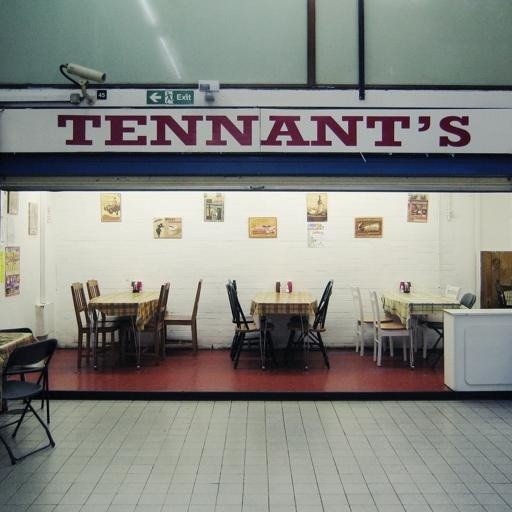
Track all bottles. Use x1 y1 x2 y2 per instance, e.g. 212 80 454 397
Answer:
286 281 293 293
131 281 142 293
399 281 411 294
275 281 280 293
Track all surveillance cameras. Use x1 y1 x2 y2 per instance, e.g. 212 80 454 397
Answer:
67 62 106 83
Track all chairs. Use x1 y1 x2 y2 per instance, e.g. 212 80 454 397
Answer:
350 284 478 370
223 276 335 370
70 276 203 371
1 327 58 464
